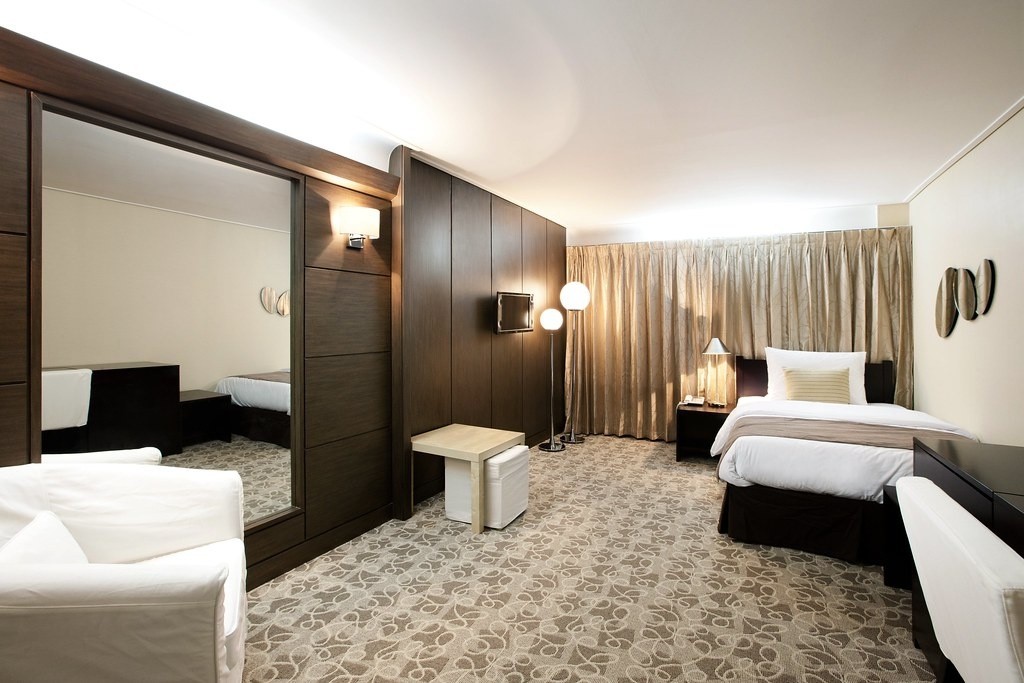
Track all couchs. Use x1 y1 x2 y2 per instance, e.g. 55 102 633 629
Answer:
0 458 249 683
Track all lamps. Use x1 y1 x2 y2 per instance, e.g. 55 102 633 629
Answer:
330 205 382 253
559 281 592 444
537 308 567 453
701 338 733 407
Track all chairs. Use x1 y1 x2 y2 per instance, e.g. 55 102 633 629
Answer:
894 475 1024 683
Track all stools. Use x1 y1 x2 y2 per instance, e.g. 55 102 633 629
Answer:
445 445 530 531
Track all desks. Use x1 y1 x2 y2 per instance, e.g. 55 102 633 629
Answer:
39 360 182 459
910 435 1024 683
409 423 526 535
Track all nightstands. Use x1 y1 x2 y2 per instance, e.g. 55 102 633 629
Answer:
675 400 735 463
180 389 232 450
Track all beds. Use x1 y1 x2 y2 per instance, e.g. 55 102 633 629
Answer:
214 369 293 450
718 355 982 566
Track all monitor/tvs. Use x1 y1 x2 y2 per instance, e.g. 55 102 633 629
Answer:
496 291 534 333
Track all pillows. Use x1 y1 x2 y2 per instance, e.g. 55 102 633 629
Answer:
784 367 852 407
762 346 868 405
